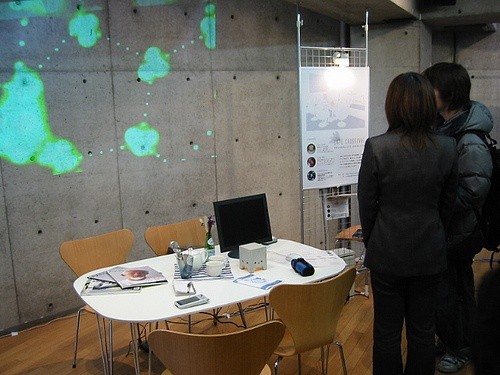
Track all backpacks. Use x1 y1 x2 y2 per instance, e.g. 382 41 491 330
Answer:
449 130 500 252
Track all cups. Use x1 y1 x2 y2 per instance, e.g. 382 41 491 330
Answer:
206 261 225 276
184 245 208 270
176 254 193 279
209 255 228 268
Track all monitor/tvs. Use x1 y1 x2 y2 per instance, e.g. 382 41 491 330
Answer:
213 193 273 259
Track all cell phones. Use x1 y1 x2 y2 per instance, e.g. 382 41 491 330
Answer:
175 294 209 309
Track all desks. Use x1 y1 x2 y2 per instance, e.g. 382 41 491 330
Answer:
73 231 346 375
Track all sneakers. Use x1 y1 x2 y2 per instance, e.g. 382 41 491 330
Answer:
438 349 472 372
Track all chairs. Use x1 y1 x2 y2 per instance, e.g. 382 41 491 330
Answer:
258 266 357 375
60 229 139 368
147 320 286 375
335 224 367 272
145 217 247 334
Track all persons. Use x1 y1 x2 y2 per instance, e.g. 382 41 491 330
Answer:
420 62 494 364
358 72 460 375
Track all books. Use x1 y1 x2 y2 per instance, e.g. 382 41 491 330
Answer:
79 266 167 296
233 274 282 290
173 281 196 296
